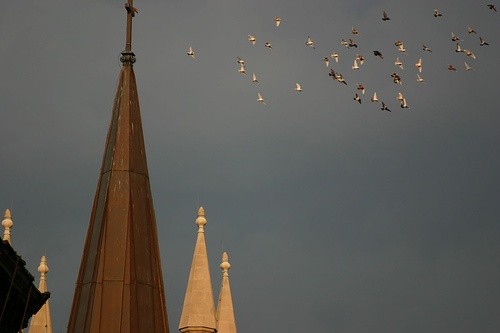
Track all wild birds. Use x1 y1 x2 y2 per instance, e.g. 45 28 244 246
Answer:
479 37 490 47
447 63 456 72
463 49 476 60
371 92 378 103
275 16 281 29
455 43 467 53
356 84 366 94
416 74 424 83
488 3 497 13
380 102 391 112
294 83 303 93
394 40 406 53
186 45 197 60
340 38 350 49
236 57 247 76
451 32 461 42
352 27 359 35
305 36 315 49
353 93 362 104
331 50 339 64
347 38 358 49
356 55 365 64
256 92 265 104
391 73 403 85
373 50 384 60
395 57 404 70
248 34 256 46
328 67 347 85
463 62 472 72
264 41 272 48
422 45 433 53
322 56 330 67
382 11 391 21
466 25 476 35
396 91 409 109
250 72 259 83
433 8 443 18
352 59 360 71
416 58 423 72
